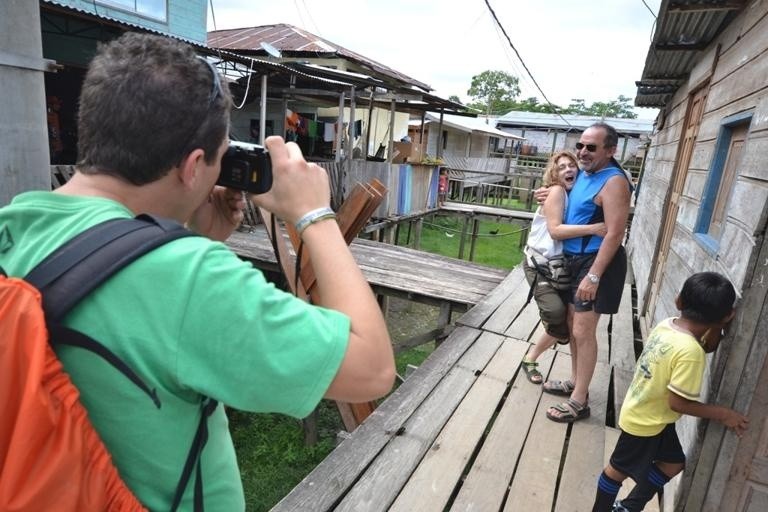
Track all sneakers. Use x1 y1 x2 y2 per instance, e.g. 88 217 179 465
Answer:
613 500 628 512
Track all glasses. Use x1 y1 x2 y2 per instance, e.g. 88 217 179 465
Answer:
575 143 596 152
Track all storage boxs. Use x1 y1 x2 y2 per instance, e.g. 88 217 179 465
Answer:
388 142 422 163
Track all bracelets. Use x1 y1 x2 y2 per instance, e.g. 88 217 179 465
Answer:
293 206 336 237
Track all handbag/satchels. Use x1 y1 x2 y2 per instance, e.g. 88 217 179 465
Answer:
548 259 571 291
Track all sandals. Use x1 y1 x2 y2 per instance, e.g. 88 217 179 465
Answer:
546 399 590 423
522 356 543 383
542 381 573 396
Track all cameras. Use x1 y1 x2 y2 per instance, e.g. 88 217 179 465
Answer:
214 139 272 194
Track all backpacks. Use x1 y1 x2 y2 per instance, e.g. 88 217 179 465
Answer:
0 215 218 512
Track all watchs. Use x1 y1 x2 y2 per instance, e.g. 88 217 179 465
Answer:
585 272 601 283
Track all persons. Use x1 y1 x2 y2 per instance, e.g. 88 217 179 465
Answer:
589 272 749 511
438 167 451 208
519 151 609 384
0 30 396 511
531 122 634 423
